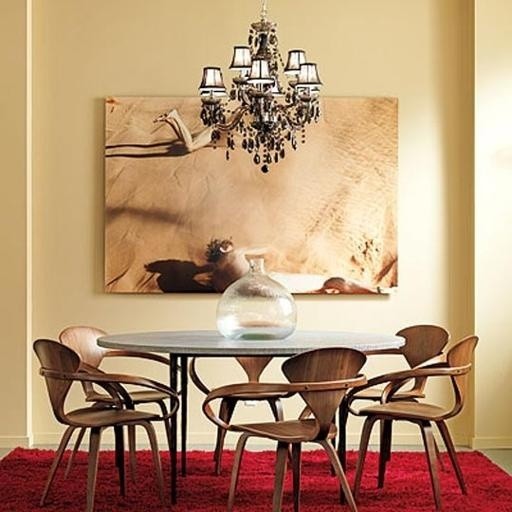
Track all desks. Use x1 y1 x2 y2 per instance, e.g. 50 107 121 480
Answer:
98 328 407 512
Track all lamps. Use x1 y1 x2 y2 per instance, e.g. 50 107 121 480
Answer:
197 1 321 164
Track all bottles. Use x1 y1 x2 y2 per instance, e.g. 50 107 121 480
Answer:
214 257 299 343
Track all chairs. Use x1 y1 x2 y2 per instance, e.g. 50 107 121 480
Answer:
189 355 297 477
58 325 186 485
202 348 367 511
299 324 451 476
337 335 480 511
33 339 180 512
